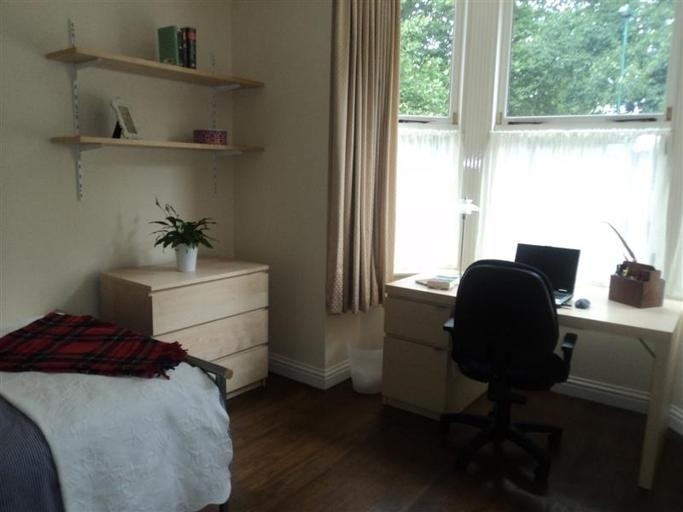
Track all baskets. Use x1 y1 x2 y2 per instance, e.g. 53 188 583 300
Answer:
193 129 227 145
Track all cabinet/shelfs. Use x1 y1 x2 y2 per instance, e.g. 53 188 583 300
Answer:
45 44 265 160
96 255 270 401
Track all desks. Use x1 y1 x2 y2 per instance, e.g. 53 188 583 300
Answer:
381 270 683 491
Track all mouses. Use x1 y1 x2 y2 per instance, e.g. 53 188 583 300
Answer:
576 297 590 308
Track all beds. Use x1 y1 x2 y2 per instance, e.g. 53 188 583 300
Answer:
0 310 232 512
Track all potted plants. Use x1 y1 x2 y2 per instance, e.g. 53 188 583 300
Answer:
149 195 219 273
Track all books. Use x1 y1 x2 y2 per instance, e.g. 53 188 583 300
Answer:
158 25 197 69
427 275 459 290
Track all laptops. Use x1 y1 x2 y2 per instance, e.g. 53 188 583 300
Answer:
514 243 579 307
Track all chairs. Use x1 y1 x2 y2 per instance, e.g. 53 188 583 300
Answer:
439 258 579 495
